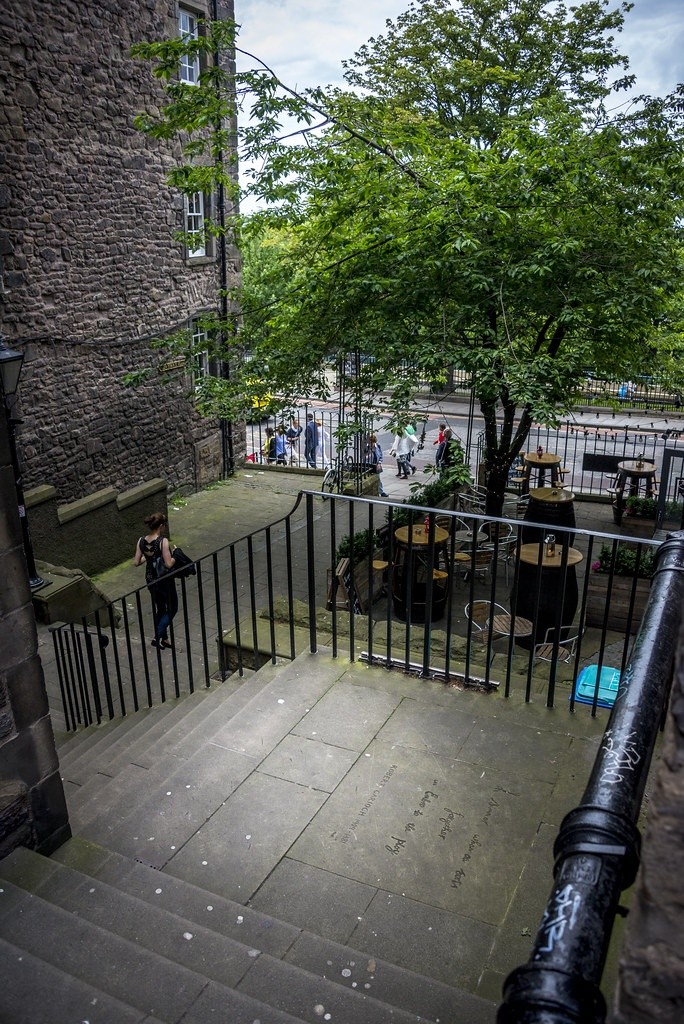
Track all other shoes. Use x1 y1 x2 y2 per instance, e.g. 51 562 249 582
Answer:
161 640 172 648
151 639 164 649
395 466 417 480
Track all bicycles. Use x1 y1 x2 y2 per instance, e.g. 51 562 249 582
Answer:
320 442 357 501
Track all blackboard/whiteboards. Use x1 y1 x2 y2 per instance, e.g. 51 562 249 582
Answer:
506 451 526 486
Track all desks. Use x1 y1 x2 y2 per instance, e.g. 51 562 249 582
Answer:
512 542 583 651
484 615 533 654
395 525 449 583
613 460 657 500
502 493 519 515
455 530 488 550
524 453 561 494
521 488 577 548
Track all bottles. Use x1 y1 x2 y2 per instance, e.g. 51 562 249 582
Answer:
636 452 644 468
425 517 429 532
537 446 543 458
545 535 556 557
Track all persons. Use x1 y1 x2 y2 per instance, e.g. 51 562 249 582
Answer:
433 423 454 479
627 380 637 396
134 512 178 650
366 436 390 497
389 420 418 479
260 414 330 469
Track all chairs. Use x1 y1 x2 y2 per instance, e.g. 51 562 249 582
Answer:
465 600 516 654
437 484 529 585
534 625 585 664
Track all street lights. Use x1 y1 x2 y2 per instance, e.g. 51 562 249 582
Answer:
0 339 48 588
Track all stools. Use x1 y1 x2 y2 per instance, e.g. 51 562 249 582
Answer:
606 474 662 497
514 466 569 490
373 549 471 580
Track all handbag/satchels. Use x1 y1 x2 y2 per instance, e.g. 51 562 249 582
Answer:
155 558 174 577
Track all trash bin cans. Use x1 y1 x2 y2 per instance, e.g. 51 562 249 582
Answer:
569 663 621 708
